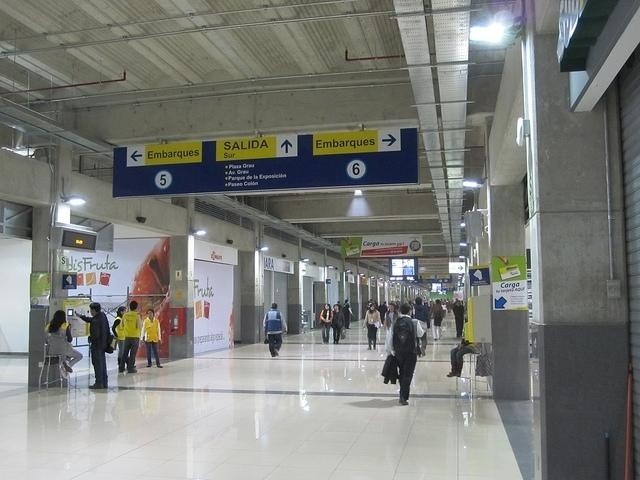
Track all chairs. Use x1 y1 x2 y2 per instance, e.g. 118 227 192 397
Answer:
39 343 71 393
454 343 493 392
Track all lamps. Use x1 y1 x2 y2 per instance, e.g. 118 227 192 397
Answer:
516 114 529 145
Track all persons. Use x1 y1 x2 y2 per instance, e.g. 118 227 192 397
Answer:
262 302 289 358
112 305 137 371
117 300 143 374
317 295 469 358
447 340 482 377
43 308 84 374
386 302 425 405
102 304 118 318
74 301 111 390
140 308 164 369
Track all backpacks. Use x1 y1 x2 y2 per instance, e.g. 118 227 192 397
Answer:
394 317 417 355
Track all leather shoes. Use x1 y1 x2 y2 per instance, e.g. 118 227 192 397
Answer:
62 360 163 389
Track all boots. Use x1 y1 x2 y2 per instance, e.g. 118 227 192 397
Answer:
457 364 463 377
447 366 457 377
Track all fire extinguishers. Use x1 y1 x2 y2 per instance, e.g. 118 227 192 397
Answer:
174 315 179 331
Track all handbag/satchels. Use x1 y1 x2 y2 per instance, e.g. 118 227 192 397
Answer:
105 333 118 353
